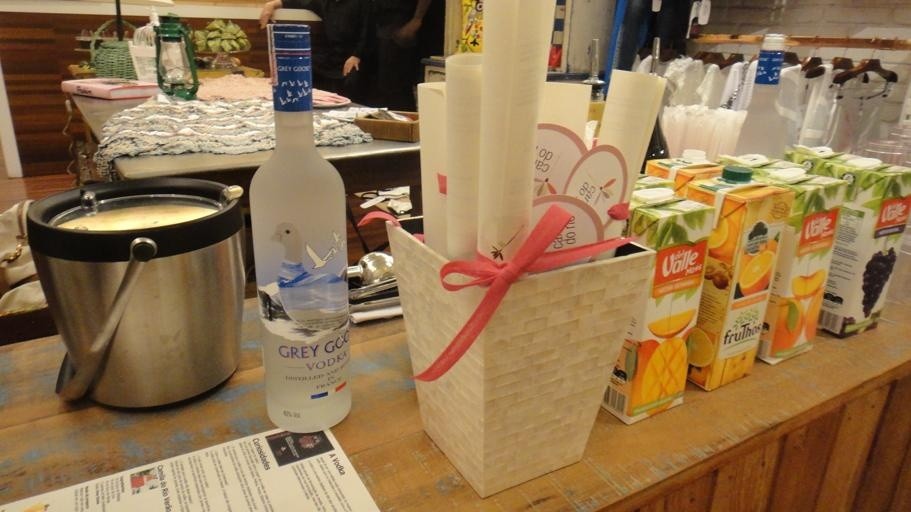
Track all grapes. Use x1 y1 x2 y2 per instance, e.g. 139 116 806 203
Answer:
863 247 897 317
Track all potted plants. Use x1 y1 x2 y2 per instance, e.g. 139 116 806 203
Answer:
188 16 253 73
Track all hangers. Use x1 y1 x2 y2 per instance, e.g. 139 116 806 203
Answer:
634 28 900 88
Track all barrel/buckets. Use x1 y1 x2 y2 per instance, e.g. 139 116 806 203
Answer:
23 178 248 413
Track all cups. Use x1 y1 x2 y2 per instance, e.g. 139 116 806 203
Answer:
864 120 911 256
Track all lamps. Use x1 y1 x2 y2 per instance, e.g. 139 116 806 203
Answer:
153 10 202 101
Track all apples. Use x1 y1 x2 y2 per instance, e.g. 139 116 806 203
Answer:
771 271 824 359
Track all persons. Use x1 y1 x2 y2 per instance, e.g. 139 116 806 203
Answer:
260 1 380 107
370 0 439 112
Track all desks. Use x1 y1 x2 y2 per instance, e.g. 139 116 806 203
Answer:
63 96 420 182
5 238 910 512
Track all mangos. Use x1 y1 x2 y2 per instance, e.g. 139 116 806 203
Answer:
627 309 692 411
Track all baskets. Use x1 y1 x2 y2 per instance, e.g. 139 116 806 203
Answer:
88 19 137 79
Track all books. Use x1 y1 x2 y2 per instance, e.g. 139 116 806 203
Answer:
62 74 163 101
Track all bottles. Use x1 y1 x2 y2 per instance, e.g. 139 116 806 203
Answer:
733 34 793 154
248 22 349 434
